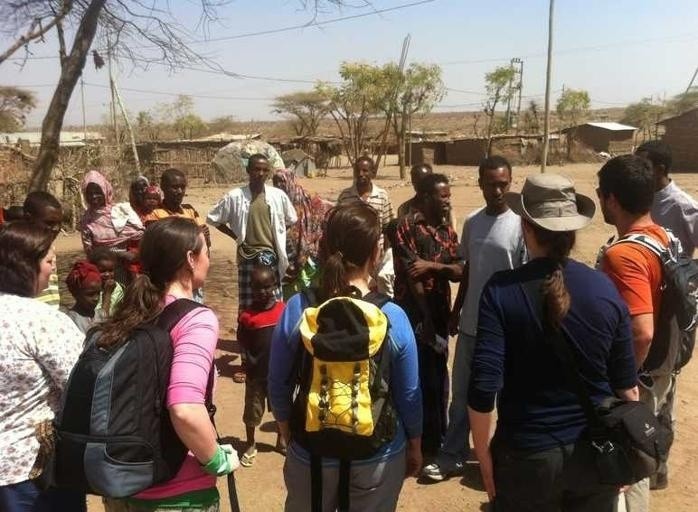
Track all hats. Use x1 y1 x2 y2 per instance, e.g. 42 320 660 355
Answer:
503 174 595 231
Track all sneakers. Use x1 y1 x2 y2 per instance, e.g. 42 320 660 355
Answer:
423 460 466 480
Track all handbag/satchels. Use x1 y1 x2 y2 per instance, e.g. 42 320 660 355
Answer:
594 401 667 484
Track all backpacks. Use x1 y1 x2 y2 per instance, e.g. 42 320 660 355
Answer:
291 285 398 512
607 228 698 389
46 298 240 512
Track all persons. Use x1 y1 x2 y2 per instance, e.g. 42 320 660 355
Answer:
209 142 698 512
2 170 241 512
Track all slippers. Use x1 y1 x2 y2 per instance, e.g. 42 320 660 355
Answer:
241 449 257 467
234 366 247 382
274 445 287 456
